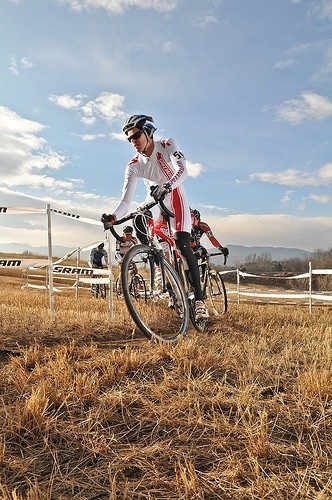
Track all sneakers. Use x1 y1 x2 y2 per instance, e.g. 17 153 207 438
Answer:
176 300 184 318
193 299 209 320
168 291 174 308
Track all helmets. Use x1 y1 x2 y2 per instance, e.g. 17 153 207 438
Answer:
122 115 158 139
98 243 104 247
123 226 133 232
192 209 200 220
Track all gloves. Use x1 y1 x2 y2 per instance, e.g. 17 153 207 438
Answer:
221 248 228 254
101 214 116 225
150 183 170 201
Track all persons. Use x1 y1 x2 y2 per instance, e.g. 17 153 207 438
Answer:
100 115 210 322
115 226 140 298
88 242 108 299
150 209 229 309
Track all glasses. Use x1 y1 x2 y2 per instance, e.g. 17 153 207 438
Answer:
127 128 146 142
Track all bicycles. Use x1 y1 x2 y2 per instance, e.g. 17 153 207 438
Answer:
115 259 147 305
191 250 228 317
103 185 206 344
90 266 109 300
